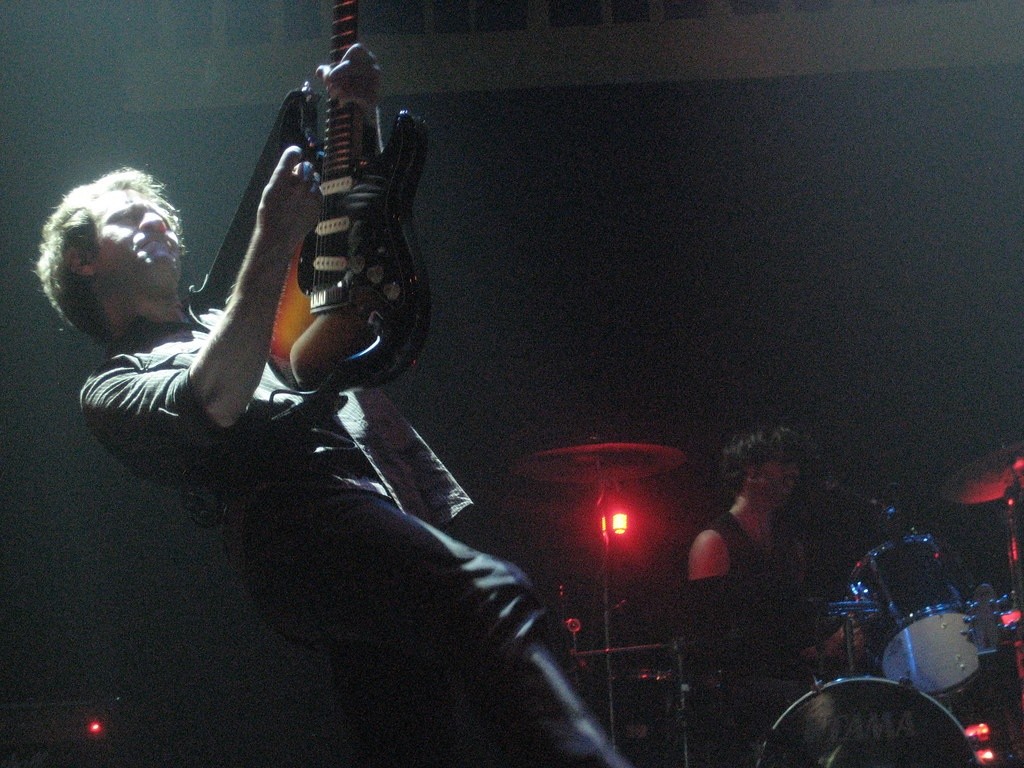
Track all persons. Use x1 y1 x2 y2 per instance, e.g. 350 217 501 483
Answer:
678 423 849 768
32 41 633 768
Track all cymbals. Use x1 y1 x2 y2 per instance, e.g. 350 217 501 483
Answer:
922 441 1024 503
527 441 687 484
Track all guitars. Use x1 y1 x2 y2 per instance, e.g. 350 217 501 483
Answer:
261 0 431 392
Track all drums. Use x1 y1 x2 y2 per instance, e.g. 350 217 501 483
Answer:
841 535 981 698
754 674 986 768
